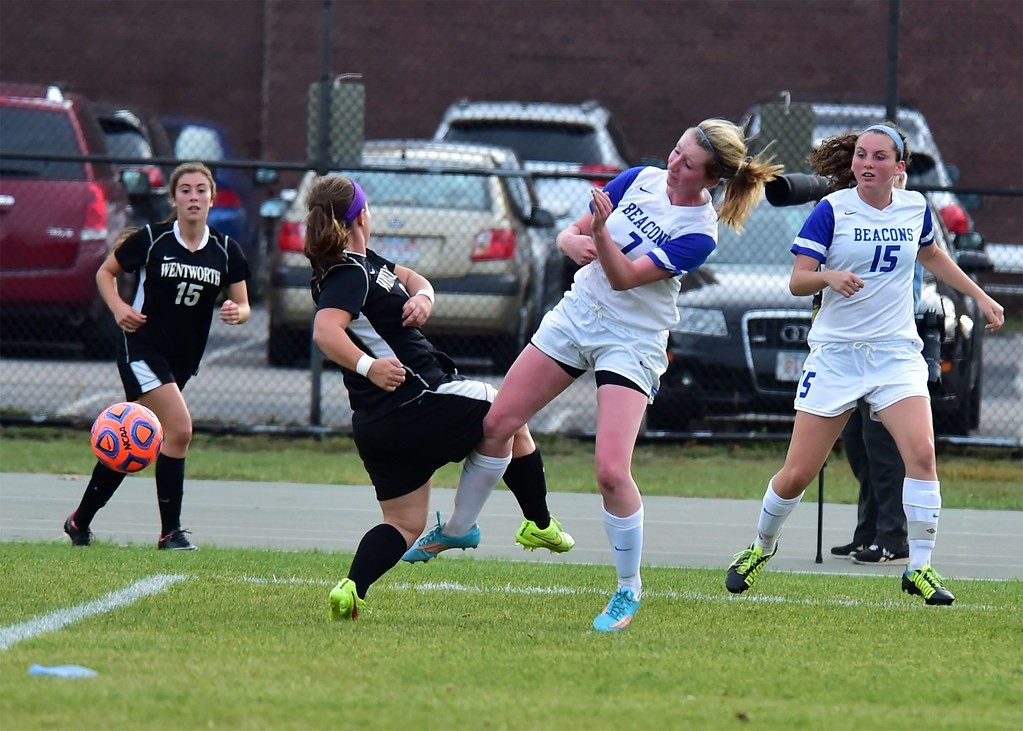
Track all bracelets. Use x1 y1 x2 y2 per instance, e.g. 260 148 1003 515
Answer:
356 354 375 377
415 289 435 304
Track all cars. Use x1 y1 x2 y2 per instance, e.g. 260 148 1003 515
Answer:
643 177 995 442
0 81 264 362
259 137 567 377
740 100 982 232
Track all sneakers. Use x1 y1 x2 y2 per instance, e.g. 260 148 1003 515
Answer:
902 569 956 606
63 512 96 547
329 579 372 623
725 539 778 593
516 514 575 555
158 527 199 552
401 511 480 564
592 589 642 632
831 542 909 565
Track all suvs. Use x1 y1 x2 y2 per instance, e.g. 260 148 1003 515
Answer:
435 97 670 261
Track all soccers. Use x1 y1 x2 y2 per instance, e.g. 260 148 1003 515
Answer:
89 401 165 475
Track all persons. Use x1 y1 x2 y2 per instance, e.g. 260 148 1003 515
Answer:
810 134 925 562
725 120 1006 605
64 165 252 551
305 177 574 620
401 117 783 631
